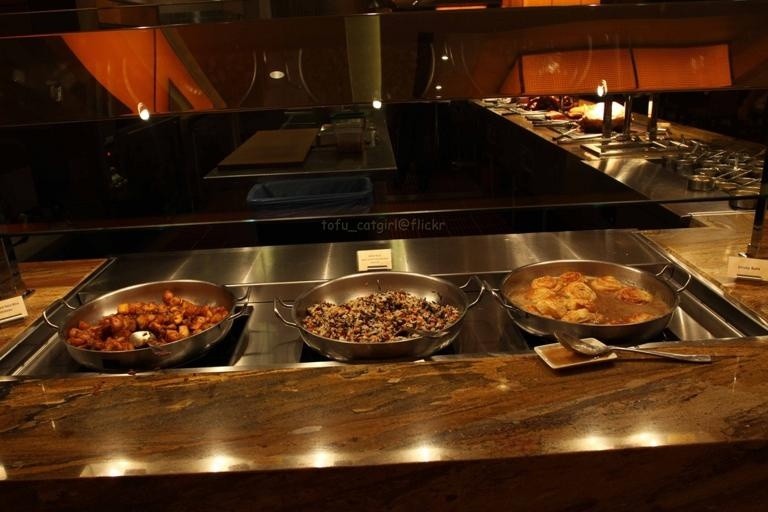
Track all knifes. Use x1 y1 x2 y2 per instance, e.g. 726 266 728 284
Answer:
557 133 603 146
552 128 577 141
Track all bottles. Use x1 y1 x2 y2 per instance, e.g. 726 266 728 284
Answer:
367 126 377 150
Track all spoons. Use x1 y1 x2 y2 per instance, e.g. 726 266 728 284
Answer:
551 329 713 365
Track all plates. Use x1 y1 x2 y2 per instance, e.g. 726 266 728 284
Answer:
533 336 618 371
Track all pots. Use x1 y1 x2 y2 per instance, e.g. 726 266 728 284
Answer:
482 262 693 344
660 144 765 211
42 277 253 373
272 270 485 363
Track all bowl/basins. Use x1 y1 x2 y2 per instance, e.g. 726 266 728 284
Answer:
581 116 625 132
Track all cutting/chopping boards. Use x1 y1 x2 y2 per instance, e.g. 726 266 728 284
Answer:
217 127 320 171
